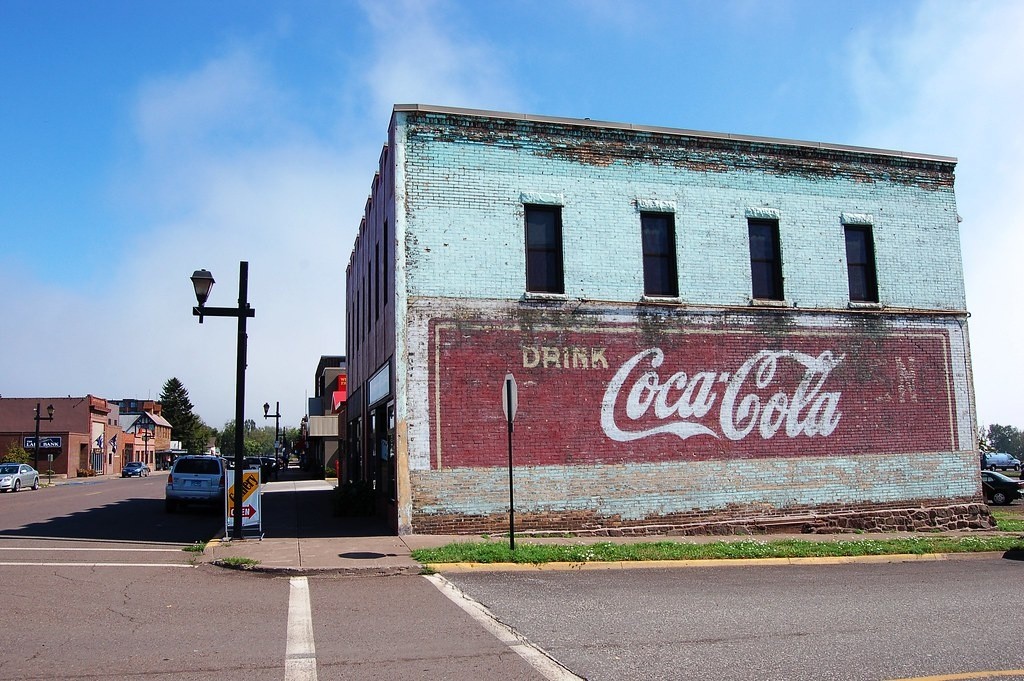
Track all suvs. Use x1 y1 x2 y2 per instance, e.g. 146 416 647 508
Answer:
164 454 230 512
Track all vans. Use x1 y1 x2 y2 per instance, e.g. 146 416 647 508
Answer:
980 450 1021 472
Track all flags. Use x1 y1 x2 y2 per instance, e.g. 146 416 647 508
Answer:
96 434 104 449
109 435 117 454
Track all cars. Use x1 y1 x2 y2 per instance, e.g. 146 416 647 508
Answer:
221 453 283 476
981 470 1024 506
0 462 40 493
121 461 151 478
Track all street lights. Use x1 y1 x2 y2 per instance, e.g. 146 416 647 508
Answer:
189 260 256 540
263 401 281 478
33 402 56 471
142 427 156 466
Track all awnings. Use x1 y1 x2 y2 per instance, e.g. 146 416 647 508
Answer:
293 438 306 450
